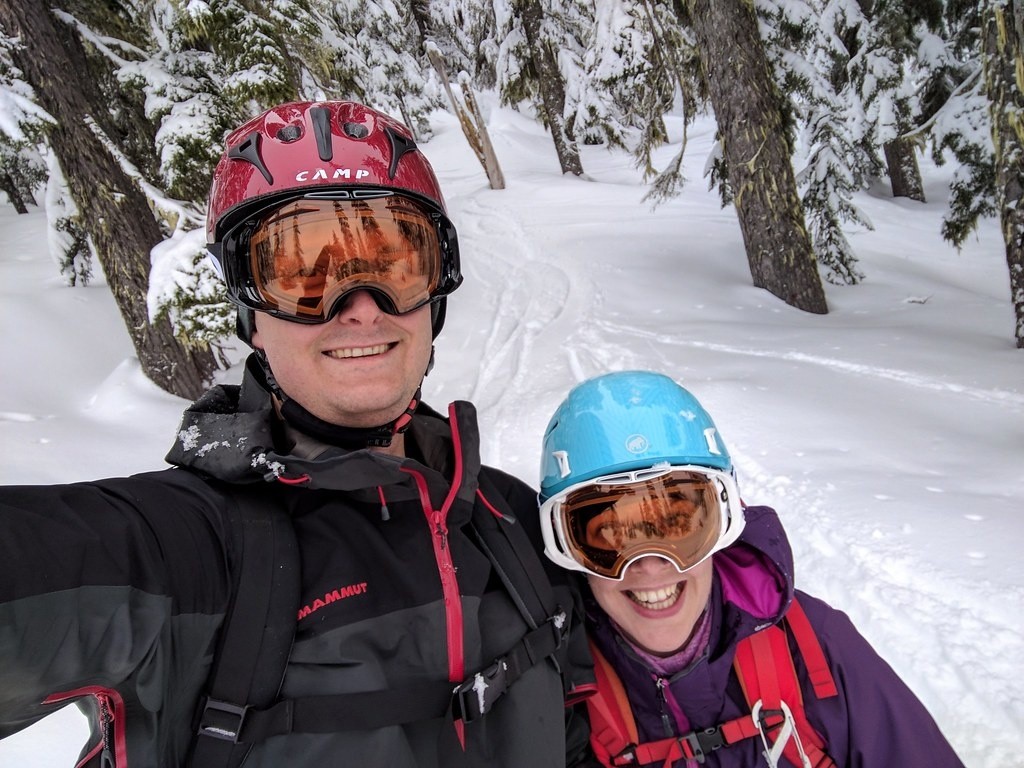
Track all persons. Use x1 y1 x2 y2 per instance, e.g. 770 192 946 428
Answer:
539 370 969 768
0 100 594 768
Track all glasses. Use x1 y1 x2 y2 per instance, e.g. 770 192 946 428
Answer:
561 470 722 581
249 189 442 325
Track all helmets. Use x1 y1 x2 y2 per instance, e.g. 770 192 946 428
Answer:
538 370 733 501
206 100 449 342
